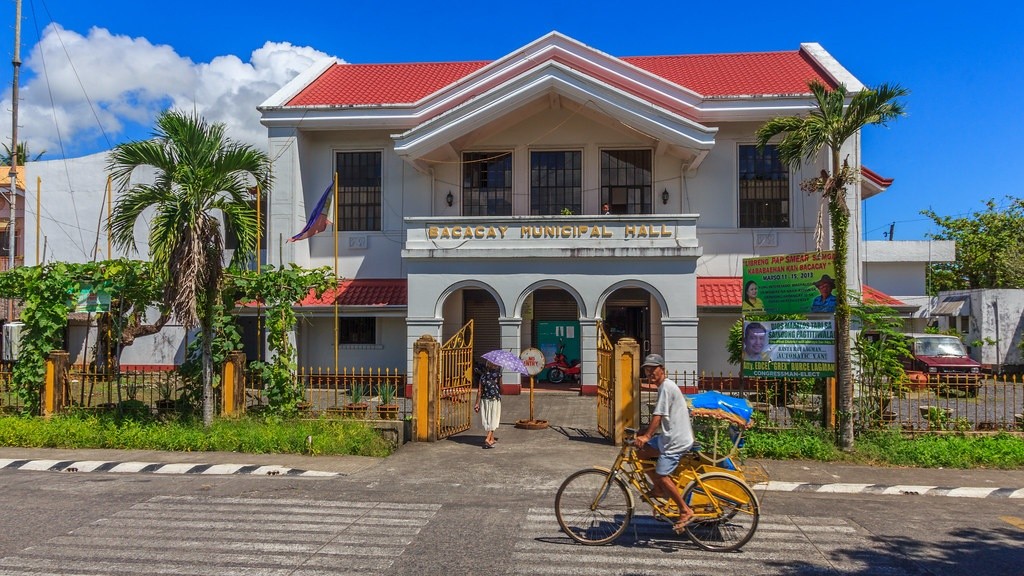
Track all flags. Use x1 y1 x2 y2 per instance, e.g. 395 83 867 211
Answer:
286 173 336 244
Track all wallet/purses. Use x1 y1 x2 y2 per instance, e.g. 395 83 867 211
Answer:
475 408 479 413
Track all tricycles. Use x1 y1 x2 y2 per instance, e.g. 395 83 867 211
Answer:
555 390 770 553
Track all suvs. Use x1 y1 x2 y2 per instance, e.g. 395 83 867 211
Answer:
881 333 982 397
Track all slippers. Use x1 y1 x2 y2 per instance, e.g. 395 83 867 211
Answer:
672 516 697 531
640 492 664 499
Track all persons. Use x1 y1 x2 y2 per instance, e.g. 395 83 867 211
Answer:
633 354 696 530
811 274 837 313
602 203 611 215
742 323 770 361
888 222 895 241
474 360 503 449
742 280 767 314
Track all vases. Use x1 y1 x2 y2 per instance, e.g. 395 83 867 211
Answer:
786 404 821 425
754 403 774 417
920 405 955 424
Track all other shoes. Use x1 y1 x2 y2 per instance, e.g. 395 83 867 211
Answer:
485 440 495 448
490 440 495 444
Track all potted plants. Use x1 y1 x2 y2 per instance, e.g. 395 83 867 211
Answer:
373 381 399 420
152 382 178 413
871 396 898 426
294 381 312 411
347 380 368 420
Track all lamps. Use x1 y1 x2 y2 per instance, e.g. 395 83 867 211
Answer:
663 187 670 204
447 190 454 207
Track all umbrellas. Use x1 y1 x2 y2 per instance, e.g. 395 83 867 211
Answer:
480 348 529 376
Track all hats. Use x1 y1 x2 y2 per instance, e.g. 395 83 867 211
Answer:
812 275 836 289
641 354 664 368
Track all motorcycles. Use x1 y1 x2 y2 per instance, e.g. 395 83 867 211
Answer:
545 340 580 383
